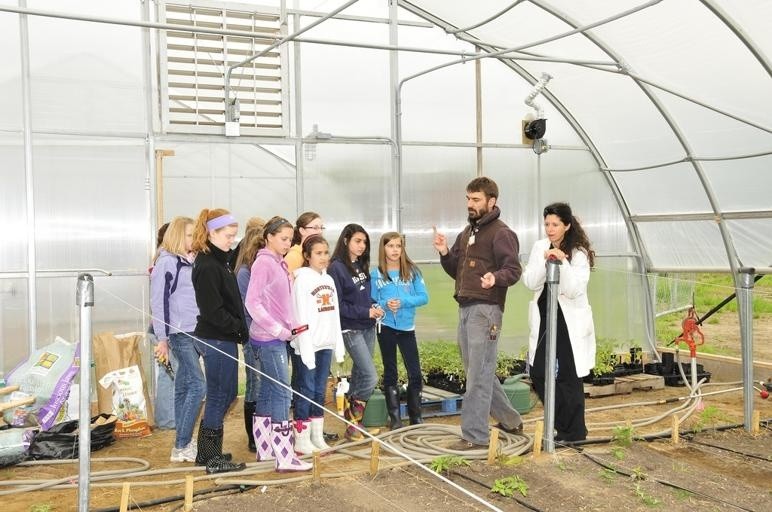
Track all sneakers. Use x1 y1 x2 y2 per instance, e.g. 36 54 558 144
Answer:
170 441 197 462
493 423 523 435
554 428 588 443
445 438 489 450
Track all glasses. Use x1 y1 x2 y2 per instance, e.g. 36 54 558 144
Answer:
304 226 325 230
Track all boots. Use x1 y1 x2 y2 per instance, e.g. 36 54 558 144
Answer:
344 393 380 445
406 387 423 424
244 400 338 472
385 386 403 430
204 426 246 473
196 419 231 465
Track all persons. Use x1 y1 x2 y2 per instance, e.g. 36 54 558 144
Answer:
149 206 428 474
519 201 596 445
431 176 524 451
